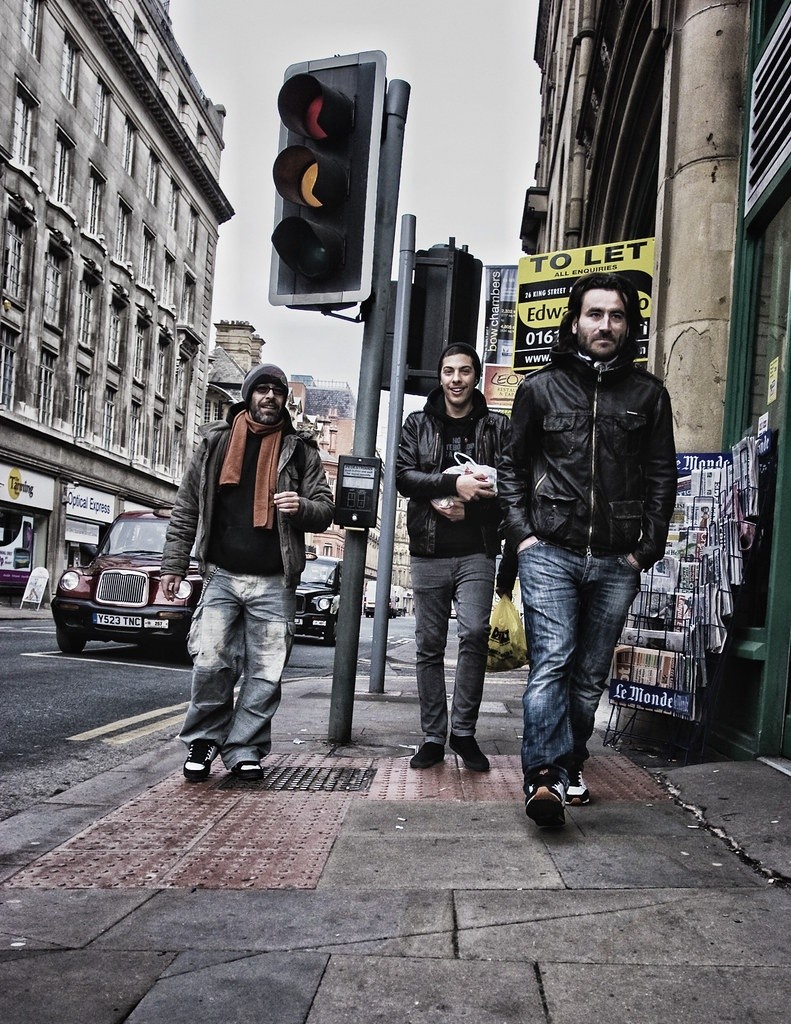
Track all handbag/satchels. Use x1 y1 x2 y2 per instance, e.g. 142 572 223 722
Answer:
486 594 528 672
431 451 498 507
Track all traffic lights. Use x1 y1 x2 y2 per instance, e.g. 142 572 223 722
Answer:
268 46 386 307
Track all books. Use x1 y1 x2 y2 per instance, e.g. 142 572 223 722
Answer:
614 435 757 720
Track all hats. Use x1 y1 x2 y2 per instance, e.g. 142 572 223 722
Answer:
438 342 481 387
241 364 289 405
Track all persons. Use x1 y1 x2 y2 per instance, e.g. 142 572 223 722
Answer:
393 345 512 773
493 275 678 828
160 366 334 780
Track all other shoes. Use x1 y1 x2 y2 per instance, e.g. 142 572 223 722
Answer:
449 731 490 770
410 742 445 768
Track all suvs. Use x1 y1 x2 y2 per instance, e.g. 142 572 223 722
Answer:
51 504 204 666
294 556 345 648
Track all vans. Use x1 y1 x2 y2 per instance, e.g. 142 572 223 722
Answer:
393 585 405 617
363 581 398 619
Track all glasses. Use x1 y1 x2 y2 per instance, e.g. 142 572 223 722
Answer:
256 384 285 395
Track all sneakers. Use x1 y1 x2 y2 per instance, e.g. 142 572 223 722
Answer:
231 761 264 781
183 739 219 779
524 767 566 827
565 754 590 805
